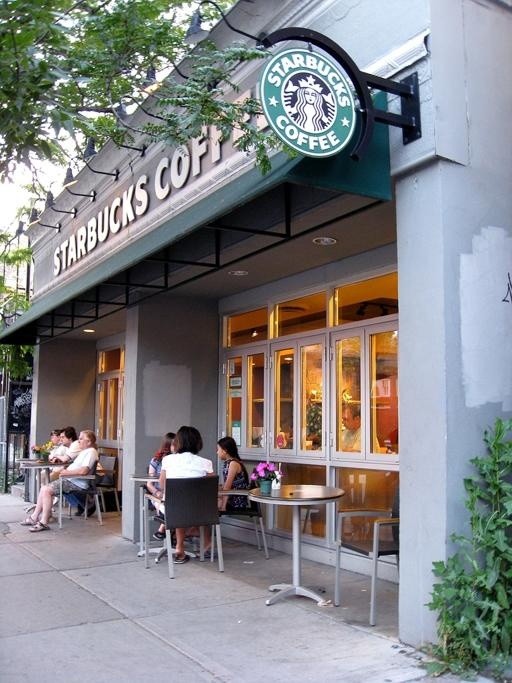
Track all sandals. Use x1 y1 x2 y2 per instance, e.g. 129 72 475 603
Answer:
20 516 37 526
29 521 50 532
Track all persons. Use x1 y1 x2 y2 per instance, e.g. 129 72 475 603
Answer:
48 426 105 517
196 435 252 558
146 432 176 541
49 428 66 444
160 426 211 565
20 429 100 532
317 403 381 454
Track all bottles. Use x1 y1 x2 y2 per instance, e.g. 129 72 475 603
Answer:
272 470 281 489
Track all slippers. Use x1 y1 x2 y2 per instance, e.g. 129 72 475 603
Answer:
171 554 190 565
194 550 216 558
151 530 166 541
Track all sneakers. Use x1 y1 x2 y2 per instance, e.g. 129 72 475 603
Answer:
87 503 96 517
74 503 84 516
